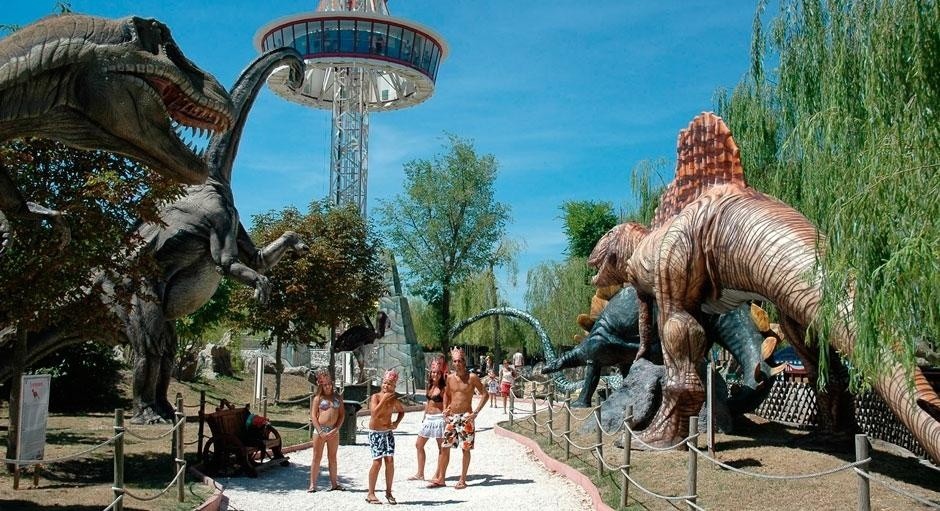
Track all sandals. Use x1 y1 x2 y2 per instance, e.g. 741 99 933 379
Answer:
426 479 446 488
408 474 424 480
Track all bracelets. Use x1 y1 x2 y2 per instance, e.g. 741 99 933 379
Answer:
473 410 478 416
318 431 322 435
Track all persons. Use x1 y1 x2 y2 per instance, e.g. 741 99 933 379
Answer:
407 357 450 483
365 367 405 506
497 359 519 416
488 373 499 407
306 367 345 494
426 346 490 490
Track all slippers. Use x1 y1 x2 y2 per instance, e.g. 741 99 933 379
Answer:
455 480 467 489
385 493 397 505
365 498 383 505
327 485 346 491
307 488 320 493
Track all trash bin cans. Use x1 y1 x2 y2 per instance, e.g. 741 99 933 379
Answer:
339 400 361 445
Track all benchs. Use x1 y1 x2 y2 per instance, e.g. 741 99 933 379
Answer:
198 398 290 478
